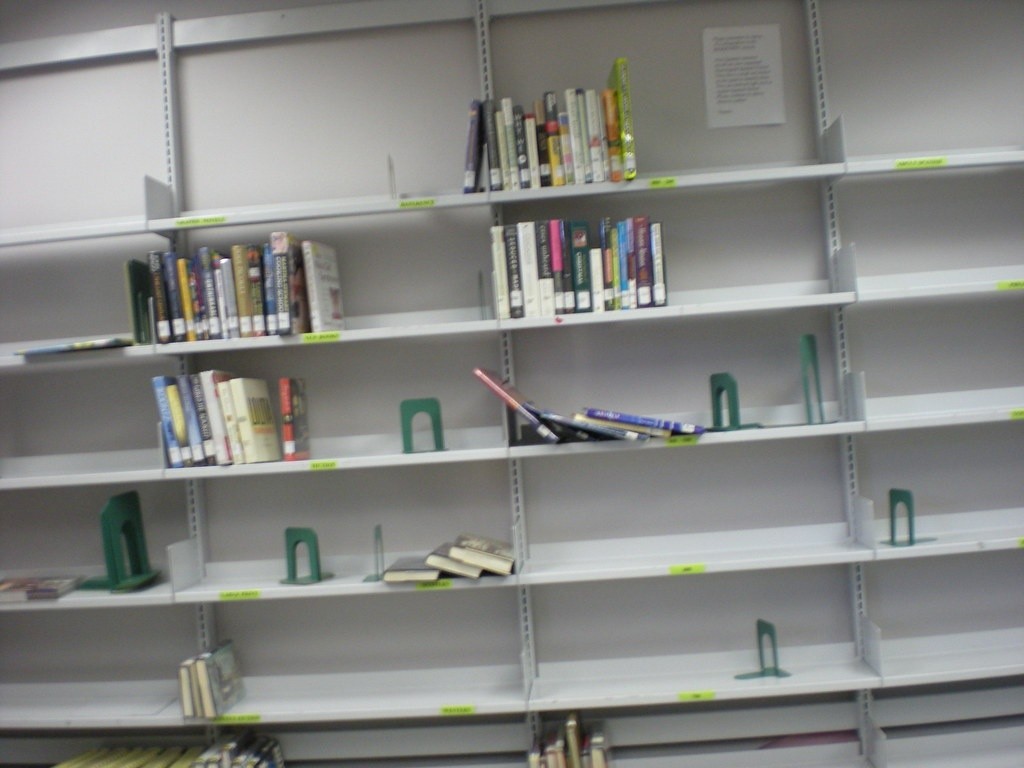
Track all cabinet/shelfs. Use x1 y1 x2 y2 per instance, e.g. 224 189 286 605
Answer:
0 0 1024 767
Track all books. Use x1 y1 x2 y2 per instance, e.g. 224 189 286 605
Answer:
473 367 706 444
0 579 80 601
53 729 286 768
383 534 515 582
152 370 307 468
14 338 132 354
528 711 610 768
180 640 247 720
463 57 637 193
490 216 667 318
124 231 344 344
